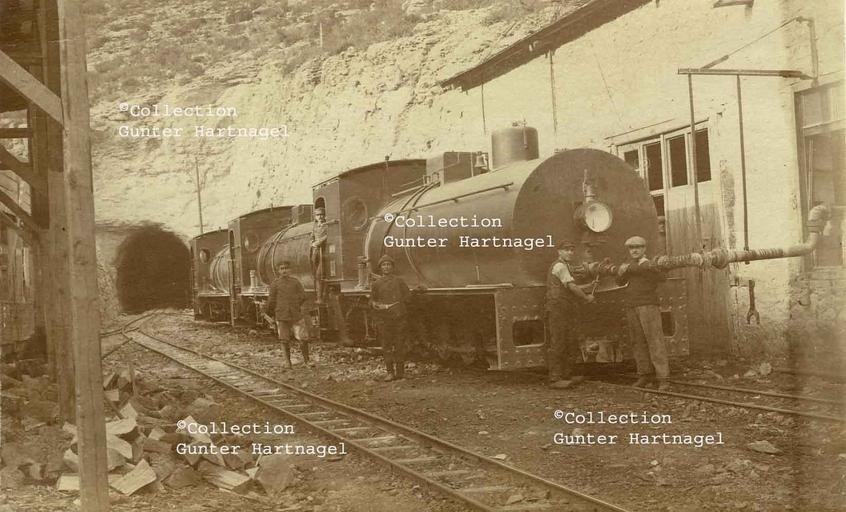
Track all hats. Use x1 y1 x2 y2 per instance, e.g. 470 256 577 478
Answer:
377 254 394 268
624 236 646 247
556 239 576 249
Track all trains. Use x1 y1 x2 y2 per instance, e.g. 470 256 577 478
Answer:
188 127 689 372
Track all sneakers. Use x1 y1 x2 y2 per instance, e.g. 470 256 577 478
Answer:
384 374 395 381
658 380 671 392
549 375 585 390
632 375 656 387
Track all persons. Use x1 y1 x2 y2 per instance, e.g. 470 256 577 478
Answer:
544 238 599 389
366 255 411 382
309 207 327 277
265 260 316 371
615 236 670 392
